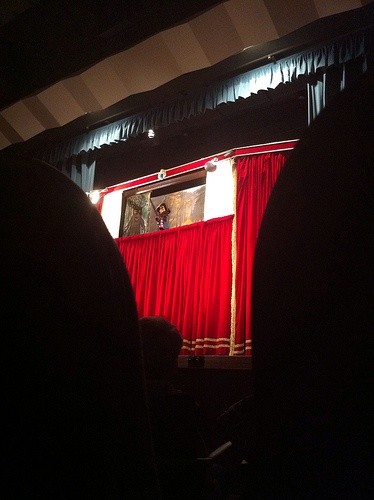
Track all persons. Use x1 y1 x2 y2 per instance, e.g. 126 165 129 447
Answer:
139 318 227 500
